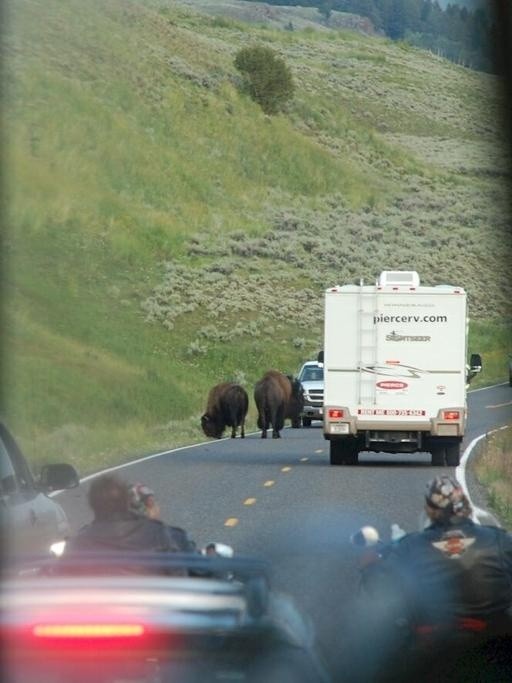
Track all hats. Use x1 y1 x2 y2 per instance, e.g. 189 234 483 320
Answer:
425 476 473 524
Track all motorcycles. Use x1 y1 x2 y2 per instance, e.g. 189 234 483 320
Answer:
355 526 511 683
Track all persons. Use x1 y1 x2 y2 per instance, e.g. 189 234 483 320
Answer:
373 475 512 651
43 474 189 576
126 482 221 579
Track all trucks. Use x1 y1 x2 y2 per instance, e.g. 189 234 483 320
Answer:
319 269 482 465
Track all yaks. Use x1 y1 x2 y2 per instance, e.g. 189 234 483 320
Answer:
252 369 306 440
198 380 251 440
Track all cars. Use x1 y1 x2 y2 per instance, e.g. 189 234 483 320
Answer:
297 360 323 427
